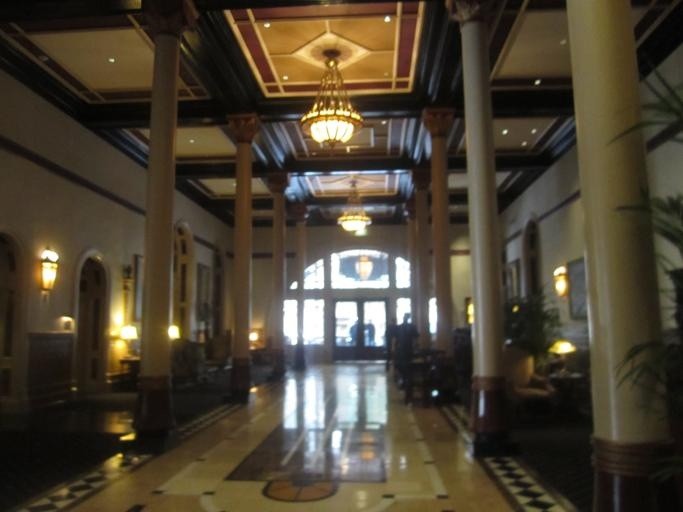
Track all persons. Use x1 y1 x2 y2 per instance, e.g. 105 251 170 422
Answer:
387 311 420 378
363 319 376 347
348 319 358 347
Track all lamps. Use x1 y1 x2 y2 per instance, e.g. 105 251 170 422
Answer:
335 178 372 238
300 48 365 151
37 248 59 300
